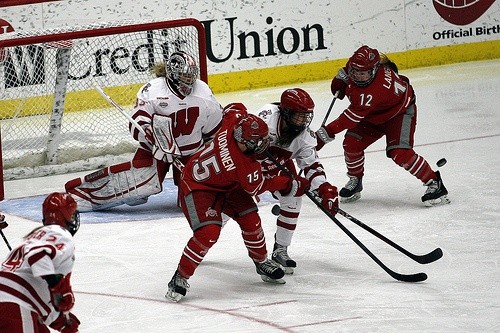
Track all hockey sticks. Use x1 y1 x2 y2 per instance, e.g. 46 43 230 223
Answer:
309 190 444 266
92 81 186 172
54 292 71 327
0 228 12 252
271 90 340 217
263 150 428 283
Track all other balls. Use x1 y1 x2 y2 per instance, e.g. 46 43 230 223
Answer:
436 158 447 167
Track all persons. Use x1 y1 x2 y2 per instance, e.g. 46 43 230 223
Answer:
318 45 450 206
0 193 81 332
163 103 311 302
221 87 339 273
66 51 224 211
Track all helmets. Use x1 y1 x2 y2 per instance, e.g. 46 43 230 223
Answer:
165 51 198 96
42 192 80 237
233 114 269 155
279 88 315 132
346 45 380 86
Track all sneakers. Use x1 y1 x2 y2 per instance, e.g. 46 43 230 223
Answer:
421 170 449 206
256 258 285 284
272 243 296 274
165 270 190 303
339 172 363 203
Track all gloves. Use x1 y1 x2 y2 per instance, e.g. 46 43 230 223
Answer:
49 311 80 333
48 272 74 312
279 175 311 197
317 182 338 216
314 126 335 151
331 67 348 100
